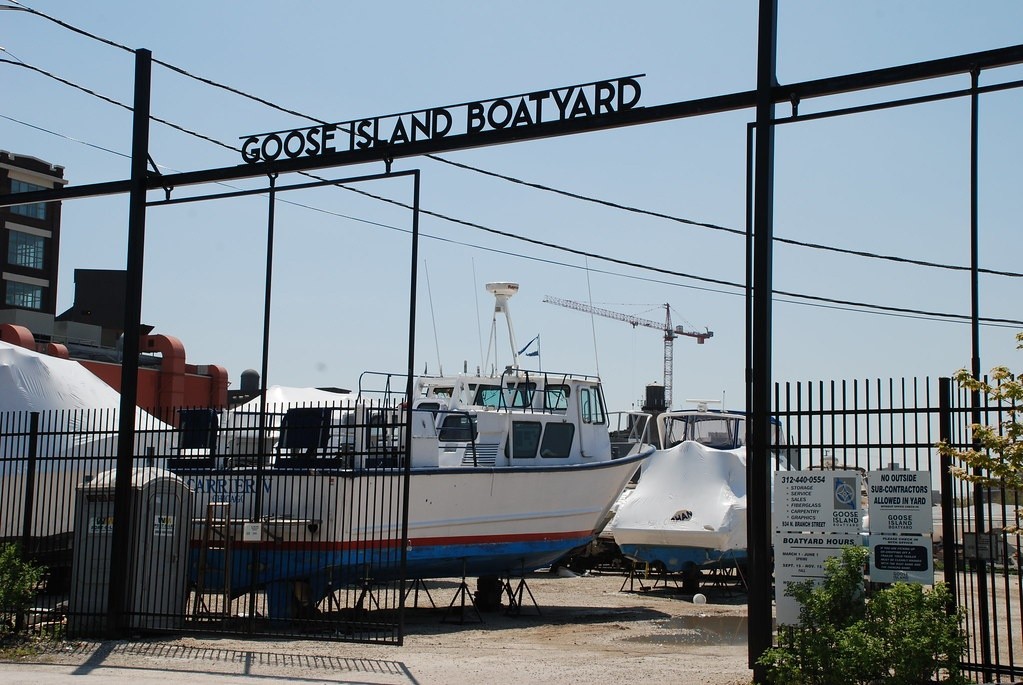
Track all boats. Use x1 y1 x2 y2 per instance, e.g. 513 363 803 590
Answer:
142 279 652 617
615 397 807 575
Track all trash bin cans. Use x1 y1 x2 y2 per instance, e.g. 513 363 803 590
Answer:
65 466 196 641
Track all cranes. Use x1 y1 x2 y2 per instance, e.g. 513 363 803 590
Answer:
540 292 713 405
